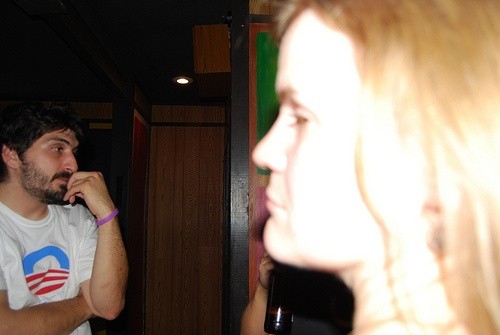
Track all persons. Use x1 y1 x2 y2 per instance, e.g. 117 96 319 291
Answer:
249 1 499 335
0 103 129 335
238 255 275 334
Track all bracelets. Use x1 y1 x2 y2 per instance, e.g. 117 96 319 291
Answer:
95 208 119 227
257 278 268 290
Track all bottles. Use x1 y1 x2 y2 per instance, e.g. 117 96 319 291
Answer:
264 270 293 335
